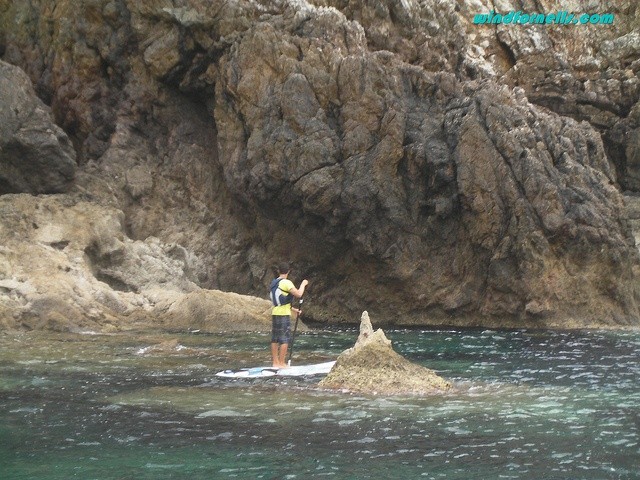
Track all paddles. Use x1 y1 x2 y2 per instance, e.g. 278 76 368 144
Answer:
287 284 307 366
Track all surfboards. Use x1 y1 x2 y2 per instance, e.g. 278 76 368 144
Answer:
216 359 338 378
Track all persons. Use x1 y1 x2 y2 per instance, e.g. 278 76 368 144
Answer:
269 263 308 368
